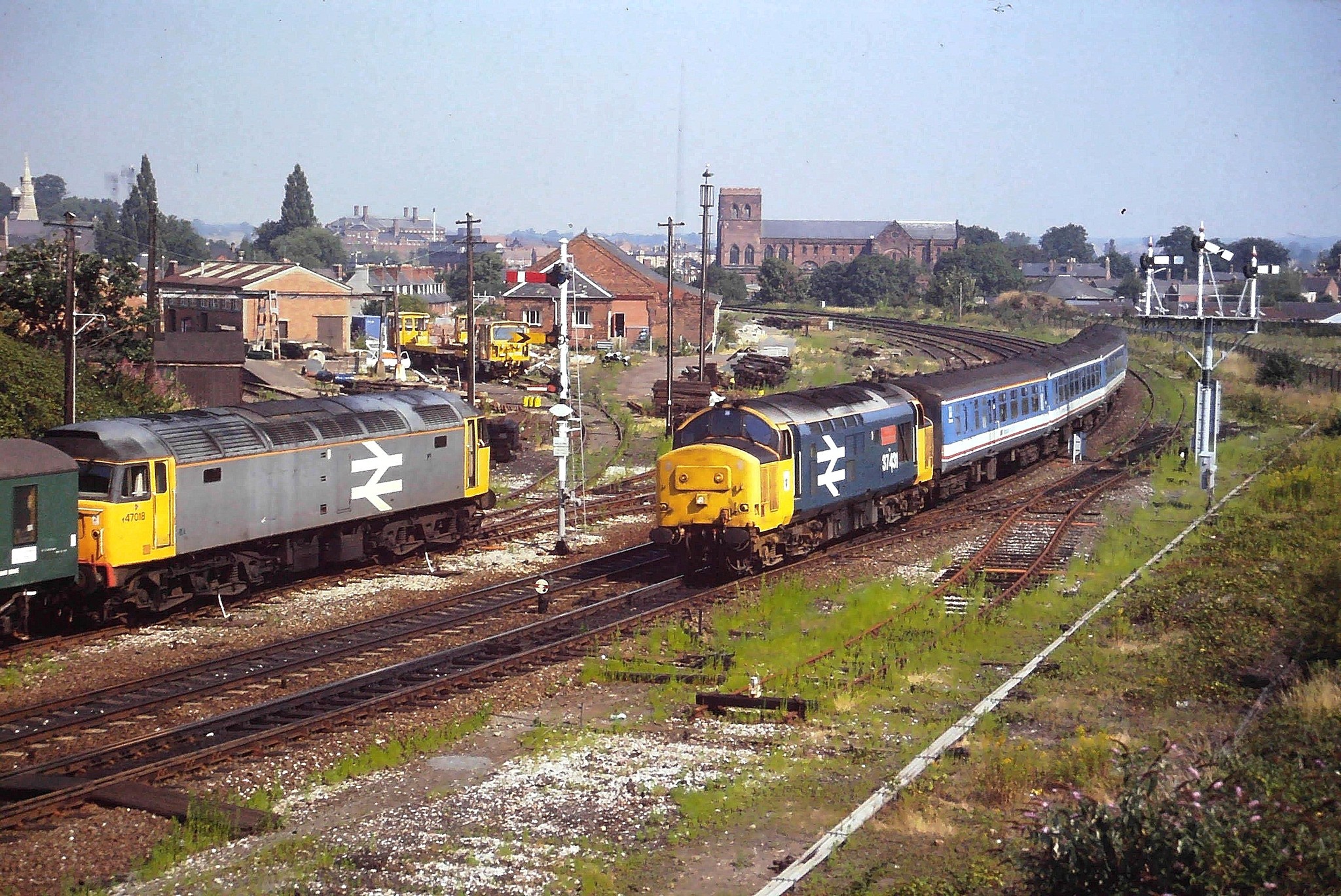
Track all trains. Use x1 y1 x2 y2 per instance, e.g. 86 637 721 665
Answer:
0 386 493 652
648 322 1126 582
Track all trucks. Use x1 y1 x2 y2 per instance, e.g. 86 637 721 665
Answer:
386 311 531 382
